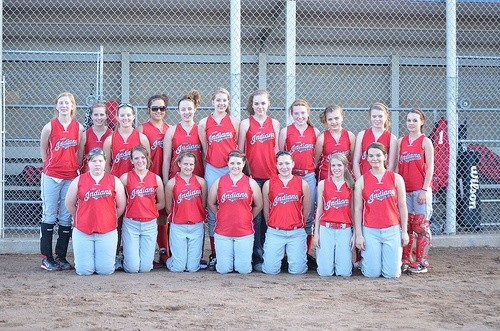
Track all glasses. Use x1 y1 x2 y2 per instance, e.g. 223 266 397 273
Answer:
276 150 290 156
150 106 167 112
118 103 133 109
89 149 104 156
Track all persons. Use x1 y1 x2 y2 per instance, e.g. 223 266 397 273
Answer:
314 104 356 271
159 151 207 273
162 91 205 260
395 108 434 273
40 92 86 271
238 90 280 264
314 153 355 277
120 144 165 274
207 150 263 274
354 142 410 279
354 102 398 270
279 99 320 254
254 151 311 274
198 87 240 271
78 102 113 177
65 147 126 275
103 103 152 269
139 93 174 267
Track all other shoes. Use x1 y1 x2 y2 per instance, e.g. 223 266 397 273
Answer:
40 257 62 271
199 259 207 269
55 256 72 269
114 255 124 270
407 264 428 272
401 263 410 272
209 254 217 271
159 248 166 266
